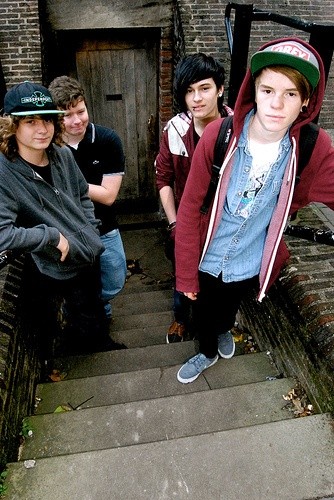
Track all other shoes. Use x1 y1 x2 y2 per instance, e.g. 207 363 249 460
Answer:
177 352 219 383
166 319 187 343
218 331 236 359
105 301 114 320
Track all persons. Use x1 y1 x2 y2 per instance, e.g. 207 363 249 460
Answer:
174 35 334 385
49 75 128 327
152 51 234 344
0 81 129 385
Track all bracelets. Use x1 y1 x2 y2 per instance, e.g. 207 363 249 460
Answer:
166 221 176 233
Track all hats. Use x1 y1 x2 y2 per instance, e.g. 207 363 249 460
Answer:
3 81 66 114
251 40 321 98
